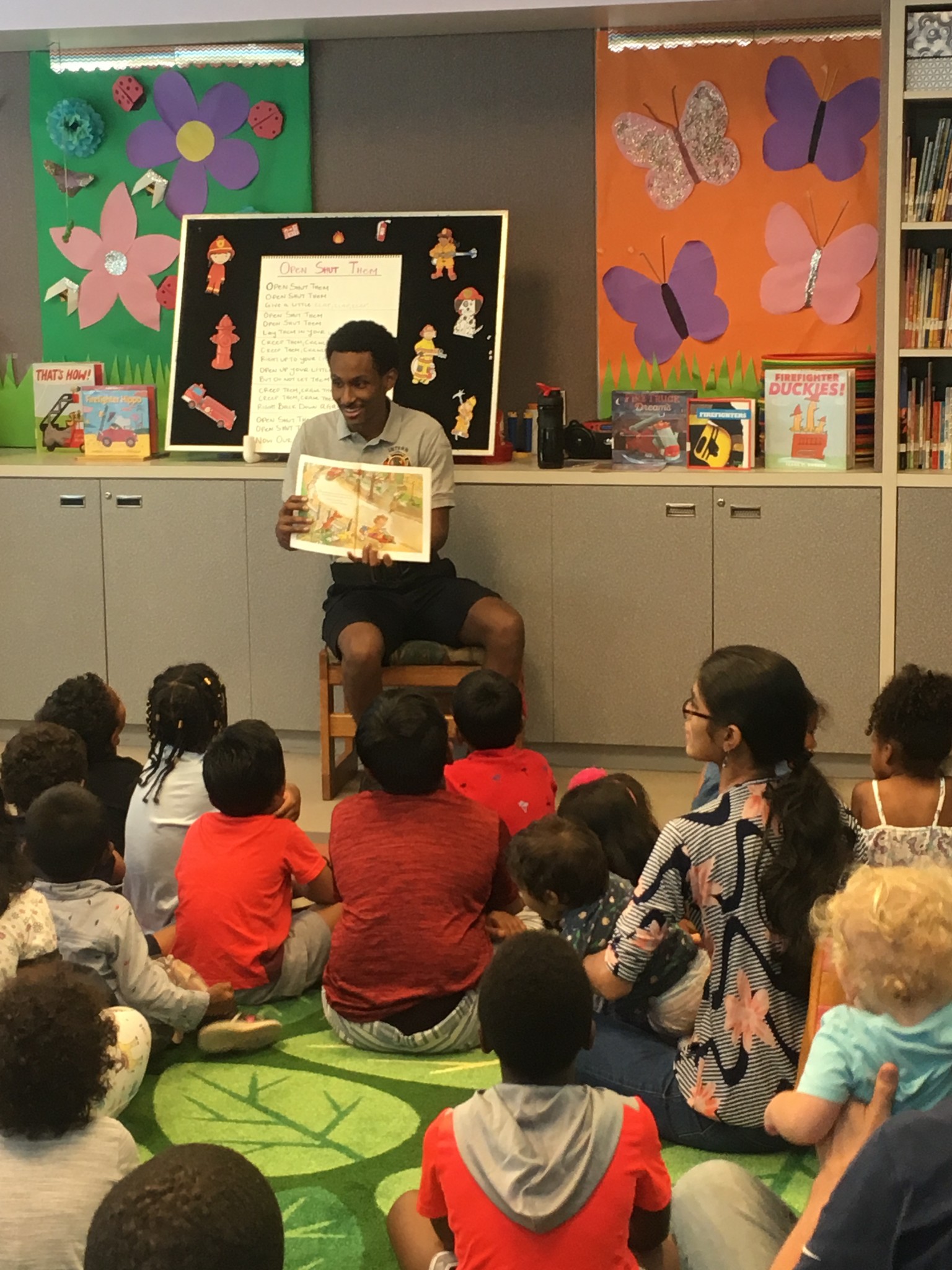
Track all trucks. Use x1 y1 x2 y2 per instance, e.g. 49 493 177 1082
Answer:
181 383 237 431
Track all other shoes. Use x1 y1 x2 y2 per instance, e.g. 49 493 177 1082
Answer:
198 1011 283 1056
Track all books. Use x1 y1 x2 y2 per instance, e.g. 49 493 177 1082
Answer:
897 99 952 471
688 398 756 471
33 363 170 463
764 366 855 473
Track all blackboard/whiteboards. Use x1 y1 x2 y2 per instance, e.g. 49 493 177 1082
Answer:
164 209 510 455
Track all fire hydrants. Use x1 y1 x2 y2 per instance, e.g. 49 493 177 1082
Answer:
209 314 241 370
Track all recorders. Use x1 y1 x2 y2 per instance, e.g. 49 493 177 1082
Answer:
566 418 613 459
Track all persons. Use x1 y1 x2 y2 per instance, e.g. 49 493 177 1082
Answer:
850 662 952 869
322 685 526 1056
0 671 287 1119
764 861 952 1145
385 929 679 1270
170 717 336 1006
83 1144 284 1270
444 667 557 835
485 644 858 1155
670 1061 952 1270
0 961 142 1270
276 321 524 791
122 663 301 935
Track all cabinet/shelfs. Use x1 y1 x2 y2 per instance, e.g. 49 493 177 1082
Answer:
0 477 884 755
886 0 952 760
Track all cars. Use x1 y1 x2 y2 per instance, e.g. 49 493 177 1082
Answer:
97 423 138 447
42 409 85 454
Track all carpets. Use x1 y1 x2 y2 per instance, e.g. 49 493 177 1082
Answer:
117 989 824 1270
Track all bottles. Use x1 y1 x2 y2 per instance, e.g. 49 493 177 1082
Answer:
535 381 563 469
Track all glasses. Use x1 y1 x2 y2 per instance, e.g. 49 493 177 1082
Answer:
682 699 712 723
